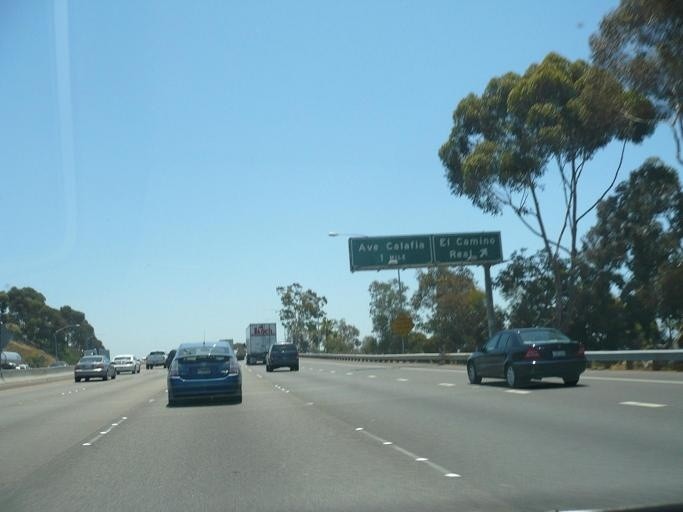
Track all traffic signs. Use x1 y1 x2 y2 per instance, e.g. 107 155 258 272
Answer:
348 231 504 273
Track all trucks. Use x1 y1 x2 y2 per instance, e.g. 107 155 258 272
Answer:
244 322 276 365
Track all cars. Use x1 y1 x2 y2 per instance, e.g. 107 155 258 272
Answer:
74 322 244 405
2 362 29 370
266 344 299 371
466 327 586 389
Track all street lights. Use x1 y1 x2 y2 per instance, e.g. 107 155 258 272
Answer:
55 324 80 363
328 232 405 352
